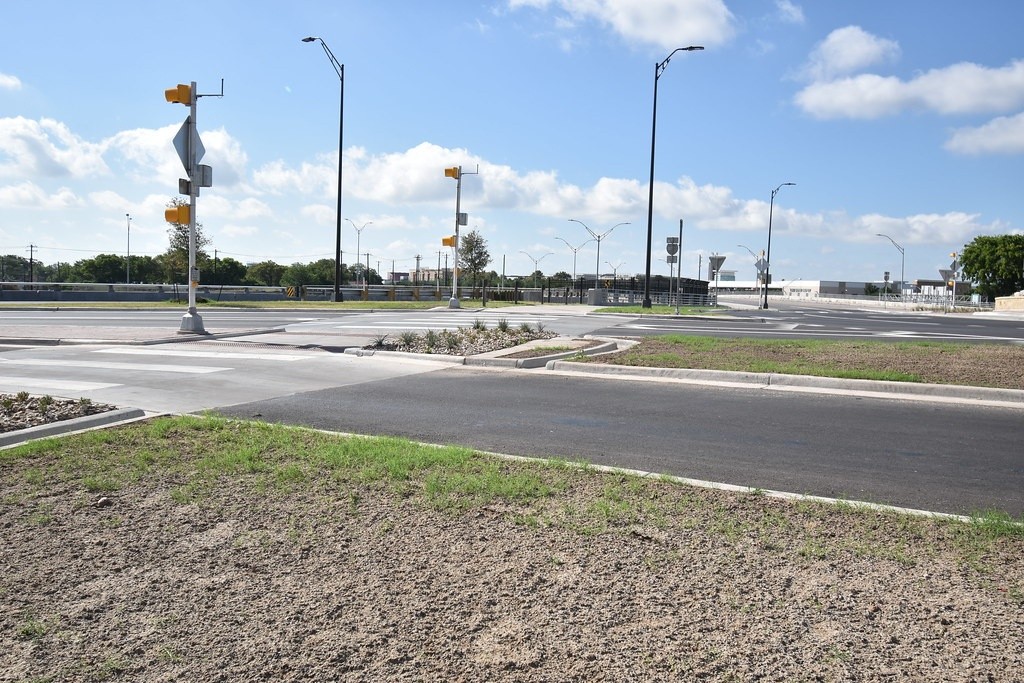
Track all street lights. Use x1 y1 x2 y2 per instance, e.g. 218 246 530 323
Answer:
519 251 555 289
568 219 632 290
555 237 597 289
345 218 373 289
641 45 705 309
605 261 626 293
762 183 797 309
125 213 132 284
301 36 345 302
876 233 904 302
737 244 759 293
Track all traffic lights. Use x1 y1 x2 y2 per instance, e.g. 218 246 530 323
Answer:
442 236 456 247
164 84 190 105
950 252 955 257
948 281 954 286
445 167 458 179
165 205 190 225
